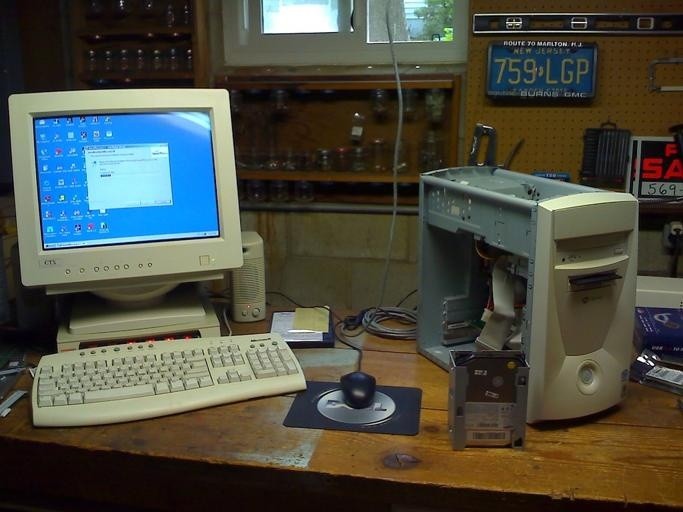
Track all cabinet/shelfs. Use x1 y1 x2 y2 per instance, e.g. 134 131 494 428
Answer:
3 4 208 88
208 65 459 206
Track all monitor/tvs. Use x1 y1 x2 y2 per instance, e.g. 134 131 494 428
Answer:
9 88 244 336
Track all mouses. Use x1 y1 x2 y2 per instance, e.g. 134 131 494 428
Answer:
340 371 376 409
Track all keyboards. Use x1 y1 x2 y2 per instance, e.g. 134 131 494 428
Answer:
32 333 308 427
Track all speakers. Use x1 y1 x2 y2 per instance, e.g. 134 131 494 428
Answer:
232 231 266 323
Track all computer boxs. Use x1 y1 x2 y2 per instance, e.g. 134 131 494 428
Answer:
416 166 638 425
